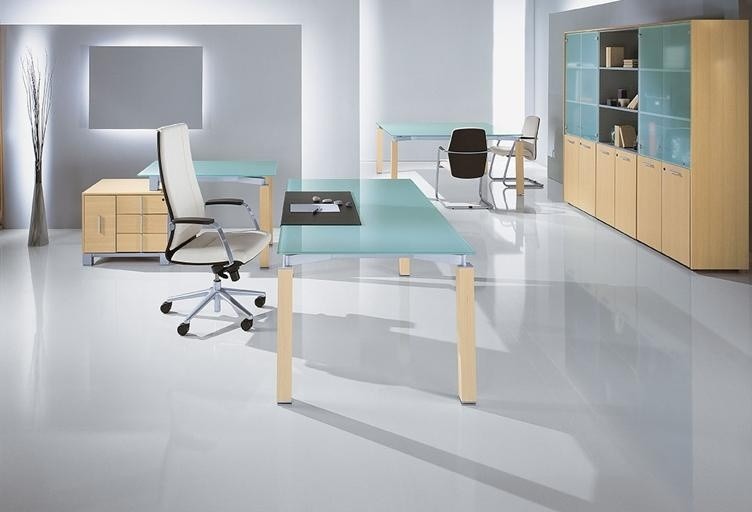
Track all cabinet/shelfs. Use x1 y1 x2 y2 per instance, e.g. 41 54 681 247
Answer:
637 21 751 167
600 23 640 152
564 135 598 217
82 179 170 264
563 27 601 139
597 143 638 238
638 155 752 271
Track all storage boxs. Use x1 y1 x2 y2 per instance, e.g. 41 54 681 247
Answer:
622 59 637 67
614 125 636 147
605 46 624 66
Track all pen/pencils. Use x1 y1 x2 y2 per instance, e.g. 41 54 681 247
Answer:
312 206 321 214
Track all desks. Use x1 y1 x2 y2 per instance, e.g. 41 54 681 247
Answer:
376 119 524 195
137 160 277 267
276 179 476 404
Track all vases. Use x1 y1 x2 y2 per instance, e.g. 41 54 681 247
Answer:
20 183 55 246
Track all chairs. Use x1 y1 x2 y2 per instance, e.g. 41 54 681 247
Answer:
435 128 493 209
157 123 271 336
489 116 544 187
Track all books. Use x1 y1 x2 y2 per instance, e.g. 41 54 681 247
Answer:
605 46 637 68
614 124 630 147
620 127 636 148
627 95 638 110
